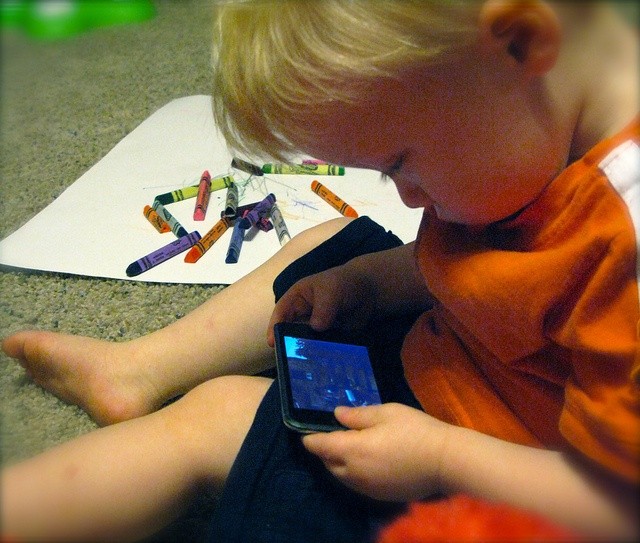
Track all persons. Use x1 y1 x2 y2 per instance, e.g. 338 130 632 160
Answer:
0 1 640 542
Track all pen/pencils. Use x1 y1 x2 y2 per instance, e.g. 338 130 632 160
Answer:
143 205 170 233
261 163 346 176
152 199 187 238
220 202 261 220
224 182 239 218
126 231 201 277
184 216 231 263
225 217 245 264
238 193 276 229
154 175 234 205
241 209 274 232
193 171 210 221
271 203 291 246
311 180 358 218
302 159 328 164
231 157 264 177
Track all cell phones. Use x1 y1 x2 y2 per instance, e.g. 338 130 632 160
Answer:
274 320 400 435
